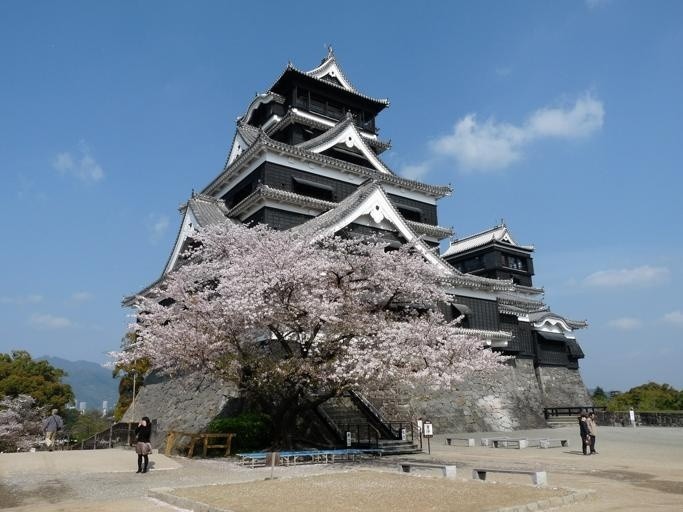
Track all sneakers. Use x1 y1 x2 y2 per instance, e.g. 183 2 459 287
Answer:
136 468 148 474
582 451 599 455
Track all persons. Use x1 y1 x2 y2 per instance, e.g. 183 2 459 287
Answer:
42 409 65 451
587 413 597 454
579 415 590 454
136 417 151 473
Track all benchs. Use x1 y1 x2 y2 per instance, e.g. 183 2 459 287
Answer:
470 466 547 486
445 436 475 447
491 439 526 449
396 459 456 482
537 437 569 448
481 437 508 447
235 447 364 470
